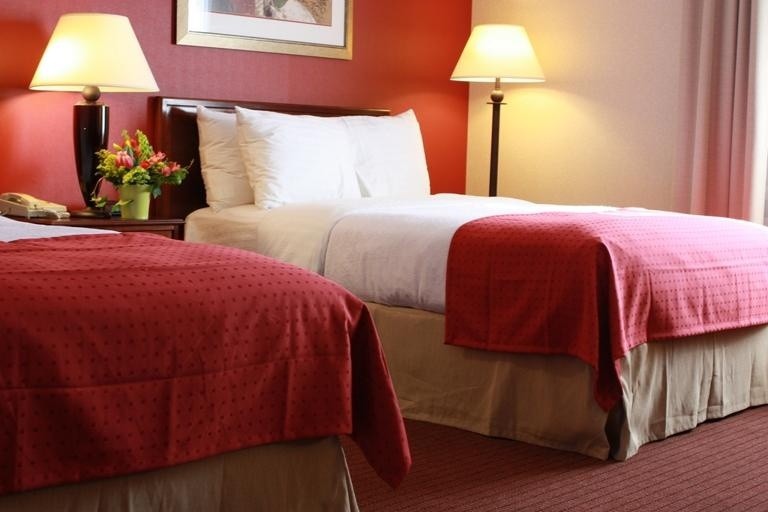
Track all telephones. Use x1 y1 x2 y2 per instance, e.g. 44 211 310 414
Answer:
0 193 70 219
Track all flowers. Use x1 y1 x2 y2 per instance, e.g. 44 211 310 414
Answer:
86 126 196 220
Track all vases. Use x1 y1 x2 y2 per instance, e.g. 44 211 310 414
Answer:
112 183 154 223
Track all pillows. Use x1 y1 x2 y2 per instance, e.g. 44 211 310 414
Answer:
230 101 361 213
194 97 260 210
321 107 431 202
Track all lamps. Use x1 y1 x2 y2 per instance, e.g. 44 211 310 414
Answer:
26 14 161 208
447 21 546 200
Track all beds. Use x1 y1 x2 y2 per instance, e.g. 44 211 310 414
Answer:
141 90 768 461
0 215 413 511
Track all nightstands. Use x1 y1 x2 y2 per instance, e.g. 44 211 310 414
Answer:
1 209 186 247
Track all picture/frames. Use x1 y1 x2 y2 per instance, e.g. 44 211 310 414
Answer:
168 0 355 63
